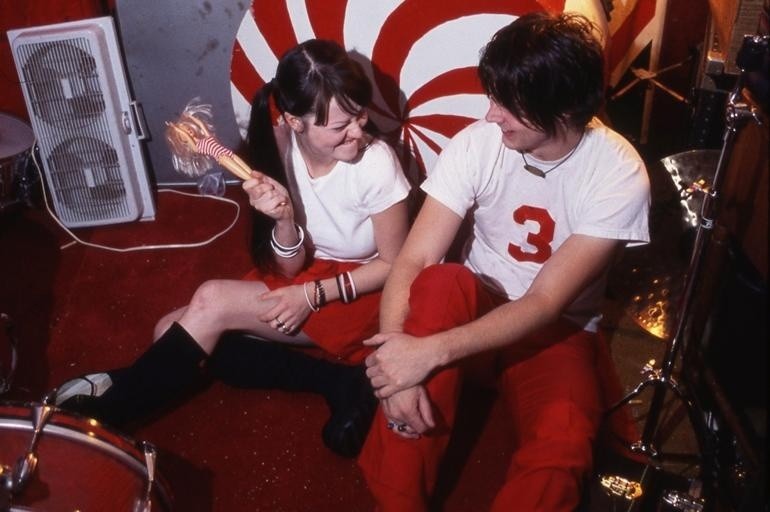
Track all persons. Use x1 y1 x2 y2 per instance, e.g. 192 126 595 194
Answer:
58 39 416 459
357 10 657 512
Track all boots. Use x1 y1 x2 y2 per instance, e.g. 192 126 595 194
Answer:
213 331 376 458
62 318 216 436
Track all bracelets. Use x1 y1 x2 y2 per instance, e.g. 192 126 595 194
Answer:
302 270 359 313
268 220 305 258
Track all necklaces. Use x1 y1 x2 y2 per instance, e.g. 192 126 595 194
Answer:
521 127 584 179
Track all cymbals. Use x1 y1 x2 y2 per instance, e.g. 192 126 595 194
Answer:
607 149 722 342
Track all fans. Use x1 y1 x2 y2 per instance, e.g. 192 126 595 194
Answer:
7 15 159 231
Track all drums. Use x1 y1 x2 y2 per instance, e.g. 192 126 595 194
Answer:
0 110 49 215
1 305 19 395
1 390 177 512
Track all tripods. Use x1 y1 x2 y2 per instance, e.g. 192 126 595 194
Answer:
603 68 752 489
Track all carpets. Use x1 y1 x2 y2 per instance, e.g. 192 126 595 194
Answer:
4 175 660 511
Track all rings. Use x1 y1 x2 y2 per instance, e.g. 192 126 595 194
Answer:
276 317 284 326
386 423 394 428
398 424 407 431
283 324 291 332
